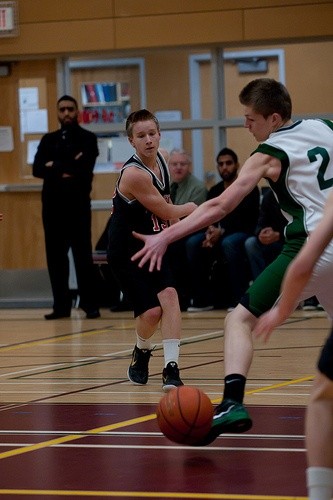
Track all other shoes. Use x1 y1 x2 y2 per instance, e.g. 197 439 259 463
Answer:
303 299 324 311
44 311 71 320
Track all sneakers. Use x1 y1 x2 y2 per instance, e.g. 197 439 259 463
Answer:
162 361 184 393
194 401 252 446
127 343 157 385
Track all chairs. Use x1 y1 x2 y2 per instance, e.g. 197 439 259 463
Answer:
74 251 108 310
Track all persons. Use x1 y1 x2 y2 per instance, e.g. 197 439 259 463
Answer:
254 190 333 500
132 78 333 446
32 95 100 320
106 109 197 391
126 147 324 310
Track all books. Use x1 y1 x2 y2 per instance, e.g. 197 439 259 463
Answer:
75 81 131 124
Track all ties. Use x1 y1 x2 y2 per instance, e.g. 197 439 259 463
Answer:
170 182 178 204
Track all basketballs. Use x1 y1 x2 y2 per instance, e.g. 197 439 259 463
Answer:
157 384 213 444
